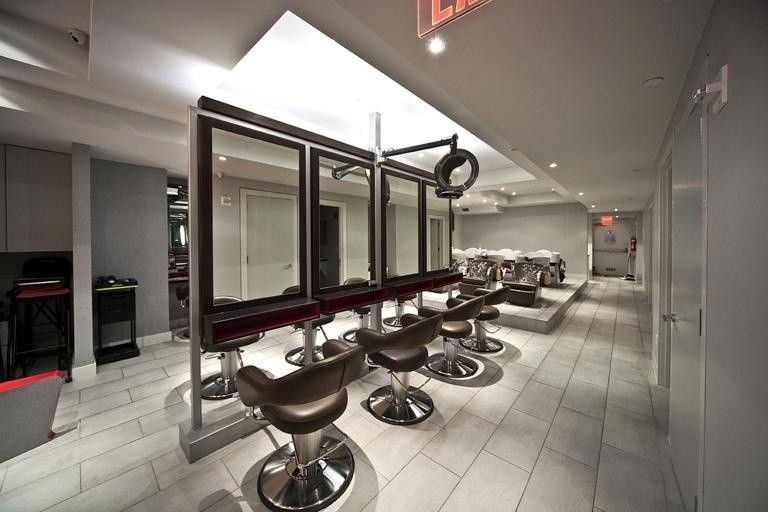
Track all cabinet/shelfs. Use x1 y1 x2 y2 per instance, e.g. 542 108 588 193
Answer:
95 284 140 366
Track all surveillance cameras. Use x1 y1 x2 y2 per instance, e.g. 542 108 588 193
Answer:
70 33 84 45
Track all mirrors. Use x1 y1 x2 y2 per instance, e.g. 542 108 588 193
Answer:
199 115 307 315
422 180 452 277
381 167 422 284
311 146 376 296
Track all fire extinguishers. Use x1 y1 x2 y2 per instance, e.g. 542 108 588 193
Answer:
631 236 636 251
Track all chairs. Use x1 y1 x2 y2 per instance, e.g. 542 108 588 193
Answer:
281 313 335 367
458 259 498 294
501 262 545 306
213 296 244 306
174 284 190 340
235 339 402 511
342 302 386 344
344 276 369 285
418 295 486 379
200 331 267 402
356 313 449 426
455 284 513 353
281 285 300 295
382 293 420 327
